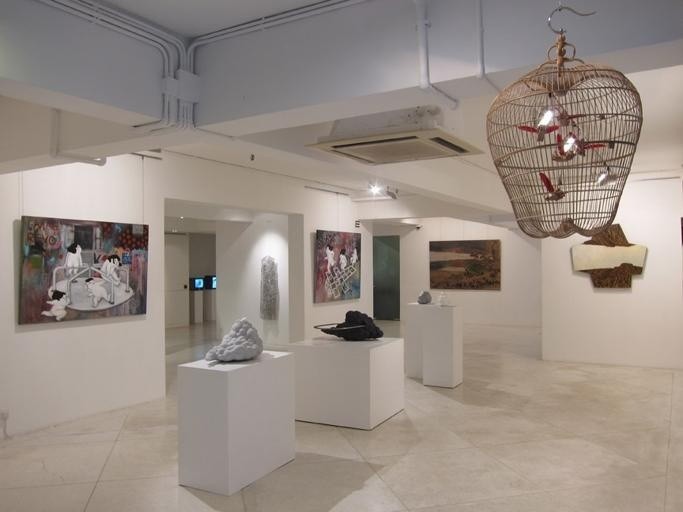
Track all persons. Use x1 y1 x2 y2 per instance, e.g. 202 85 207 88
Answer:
41 242 121 321
325 245 359 274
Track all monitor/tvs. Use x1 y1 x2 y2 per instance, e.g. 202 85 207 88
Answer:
190 278 205 290
205 275 216 289
